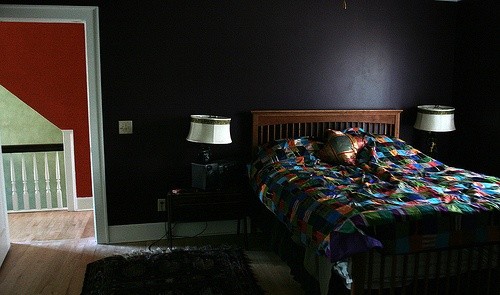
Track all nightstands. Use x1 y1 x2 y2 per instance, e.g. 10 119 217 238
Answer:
167 185 255 245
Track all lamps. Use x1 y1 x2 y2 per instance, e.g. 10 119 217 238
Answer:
415 106 456 159
184 114 235 190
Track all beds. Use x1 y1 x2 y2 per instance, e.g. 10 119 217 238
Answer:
248 106 500 295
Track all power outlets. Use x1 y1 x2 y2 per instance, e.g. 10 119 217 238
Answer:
158 199 168 211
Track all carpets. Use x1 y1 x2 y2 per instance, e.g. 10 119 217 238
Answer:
80 245 264 295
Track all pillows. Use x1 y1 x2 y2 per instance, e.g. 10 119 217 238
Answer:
356 132 392 160
320 127 370 165
